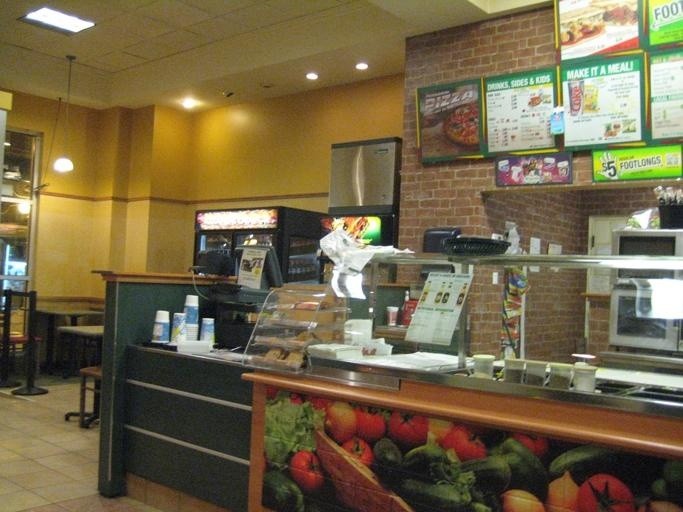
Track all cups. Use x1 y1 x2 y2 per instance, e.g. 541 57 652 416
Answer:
658 202 683 229
504 359 597 395
386 305 399 326
471 354 495 379
567 80 583 117
150 295 216 343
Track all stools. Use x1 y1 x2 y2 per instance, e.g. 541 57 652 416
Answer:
77 365 101 429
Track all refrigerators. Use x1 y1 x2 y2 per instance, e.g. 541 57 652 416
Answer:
318 214 398 288
193 206 328 283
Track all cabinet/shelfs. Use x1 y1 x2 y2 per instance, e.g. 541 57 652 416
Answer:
237 281 352 379
238 248 683 512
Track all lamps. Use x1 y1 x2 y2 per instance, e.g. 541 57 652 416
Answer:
50 53 77 175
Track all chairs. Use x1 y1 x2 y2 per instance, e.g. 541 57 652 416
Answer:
0 288 45 384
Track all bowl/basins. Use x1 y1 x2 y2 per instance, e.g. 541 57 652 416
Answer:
441 237 511 254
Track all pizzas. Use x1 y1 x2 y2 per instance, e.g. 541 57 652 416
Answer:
444 103 479 149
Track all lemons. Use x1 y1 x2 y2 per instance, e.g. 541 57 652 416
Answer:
546 471 579 512
500 490 545 512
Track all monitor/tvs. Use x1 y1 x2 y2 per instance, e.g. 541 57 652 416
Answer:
235 245 283 288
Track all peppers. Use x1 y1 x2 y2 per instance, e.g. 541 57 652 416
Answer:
490 438 547 500
262 472 304 512
548 445 619 484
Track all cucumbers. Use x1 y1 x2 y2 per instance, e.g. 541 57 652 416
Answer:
374 438 511 512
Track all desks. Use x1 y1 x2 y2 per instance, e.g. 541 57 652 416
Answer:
54 323 104 429
16 302 105 382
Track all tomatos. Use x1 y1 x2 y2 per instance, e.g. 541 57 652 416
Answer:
288 394 385 491
637 501 682 512
440 424 487 462
577 474 635 512
513 432 551 460
389 411 428 444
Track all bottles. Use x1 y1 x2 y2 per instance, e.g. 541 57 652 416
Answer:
287 235 318 282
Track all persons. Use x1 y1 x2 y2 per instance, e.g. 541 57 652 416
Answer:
442 291 450 304
420 291 429 302
457 292 464 305
434 291 443 302
